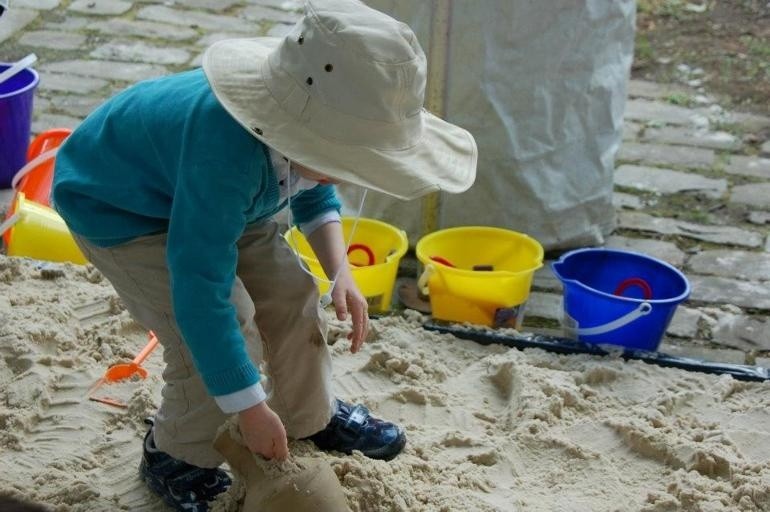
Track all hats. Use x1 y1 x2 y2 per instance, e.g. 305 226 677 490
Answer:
203 2 479 202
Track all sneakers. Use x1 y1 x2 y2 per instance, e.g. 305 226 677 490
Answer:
302 402 406 460
138 418 231 512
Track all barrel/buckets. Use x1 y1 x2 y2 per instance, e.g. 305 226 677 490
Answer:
3 128 72 246
415 226 545 332
0 191 89 265
284 215 409 312
551 246 691 351
0 53 40 189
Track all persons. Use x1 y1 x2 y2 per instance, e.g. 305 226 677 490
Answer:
49 0 479 512
